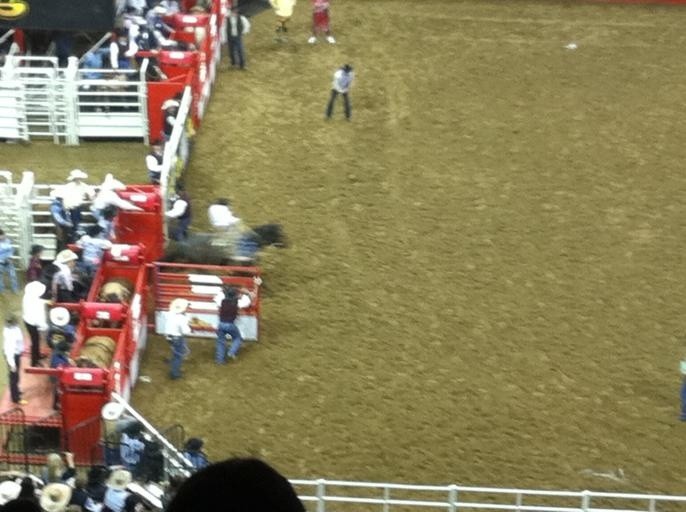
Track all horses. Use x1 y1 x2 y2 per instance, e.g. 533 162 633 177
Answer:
157 221 292 290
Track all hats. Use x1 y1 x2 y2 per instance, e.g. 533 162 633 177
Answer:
170 298 188 314
24 250 78 327
1 402 133 511
66 170 88 181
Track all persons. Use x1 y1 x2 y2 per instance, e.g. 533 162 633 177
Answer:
323 63 355 123
308 0 336 44
0 420 305 512
1 0 250 186
1 168 263 412
